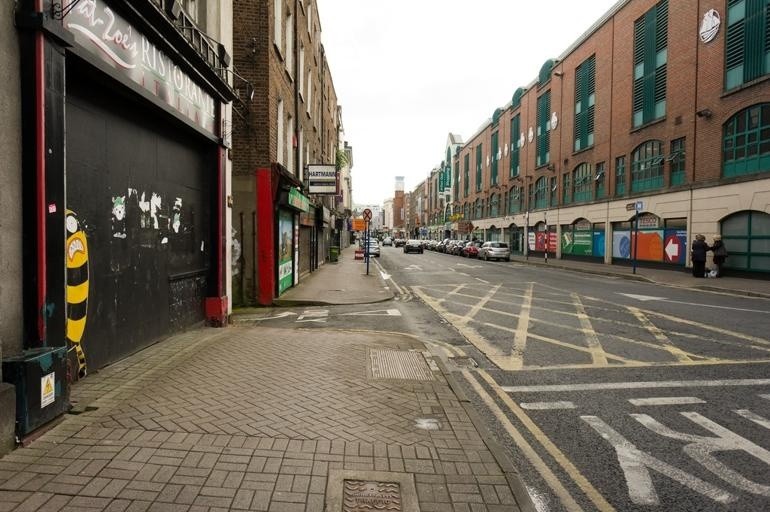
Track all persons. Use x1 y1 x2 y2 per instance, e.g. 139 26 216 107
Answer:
691 233 728 278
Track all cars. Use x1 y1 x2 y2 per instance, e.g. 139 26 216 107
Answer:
356 232 380 257
382 236 511 262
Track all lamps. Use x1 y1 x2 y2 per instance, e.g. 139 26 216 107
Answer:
161 0 183 23
177 17 234 69
208 60 255 105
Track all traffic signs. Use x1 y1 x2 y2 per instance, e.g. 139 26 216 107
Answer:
626 203 635 210
637 202 642 210
